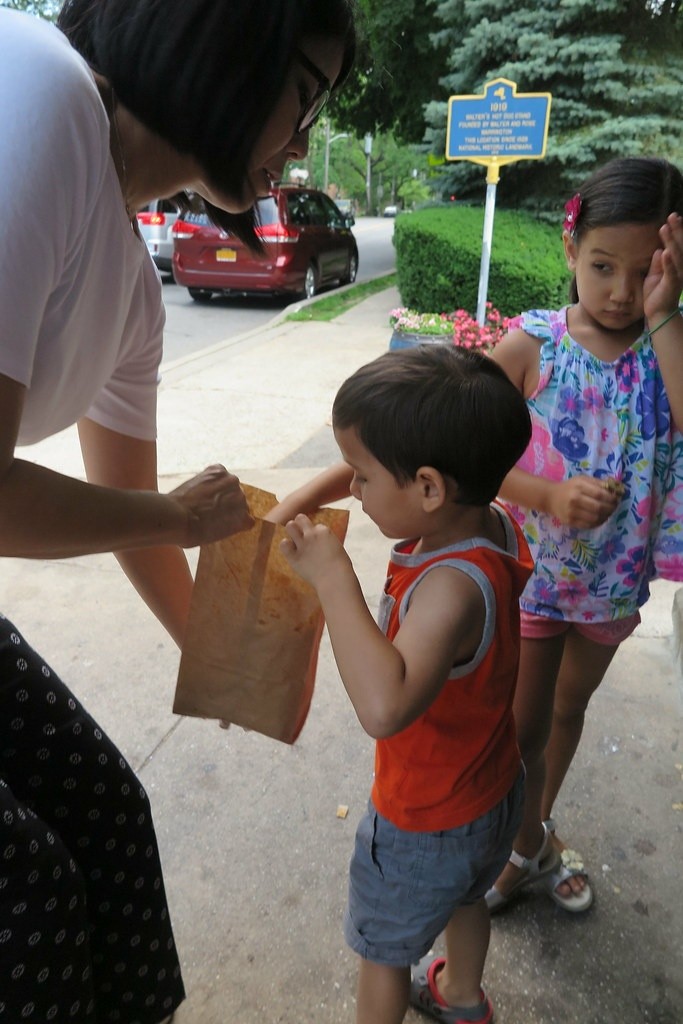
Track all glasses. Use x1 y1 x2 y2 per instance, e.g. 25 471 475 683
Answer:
283 57 334 133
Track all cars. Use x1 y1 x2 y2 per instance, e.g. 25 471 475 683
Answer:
331 199 356 221
384 205 400 217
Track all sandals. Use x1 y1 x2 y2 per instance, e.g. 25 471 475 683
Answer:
408 953 497 1024
485 822 563 916
533 818 595 911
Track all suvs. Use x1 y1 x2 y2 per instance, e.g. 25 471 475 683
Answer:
171 181 359 304
136 197 180 277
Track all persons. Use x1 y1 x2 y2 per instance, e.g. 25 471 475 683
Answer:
0 0 356 1024
481 159 683 911
264 344 534 1024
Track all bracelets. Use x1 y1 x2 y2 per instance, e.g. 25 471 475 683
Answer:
647 308 678 335
